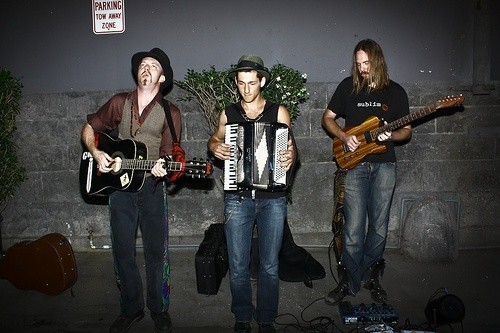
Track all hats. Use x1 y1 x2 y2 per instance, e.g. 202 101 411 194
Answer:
131 47 173 96
228 54 271 86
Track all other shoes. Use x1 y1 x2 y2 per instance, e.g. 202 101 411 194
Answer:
151 311 173 330
234 320 252 333
325 283 351 305
363 278 388 304
259 322 277 333
109 310 145 333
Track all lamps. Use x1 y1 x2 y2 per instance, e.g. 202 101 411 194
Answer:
425 288 466 326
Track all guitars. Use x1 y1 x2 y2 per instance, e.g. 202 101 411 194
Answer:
333 93 466 171
79 130 214 199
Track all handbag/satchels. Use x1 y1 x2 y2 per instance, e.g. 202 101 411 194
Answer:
194 222 229 295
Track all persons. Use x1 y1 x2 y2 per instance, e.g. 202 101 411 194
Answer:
322 38 411 306
208 55 301 333
81 48 182 333
